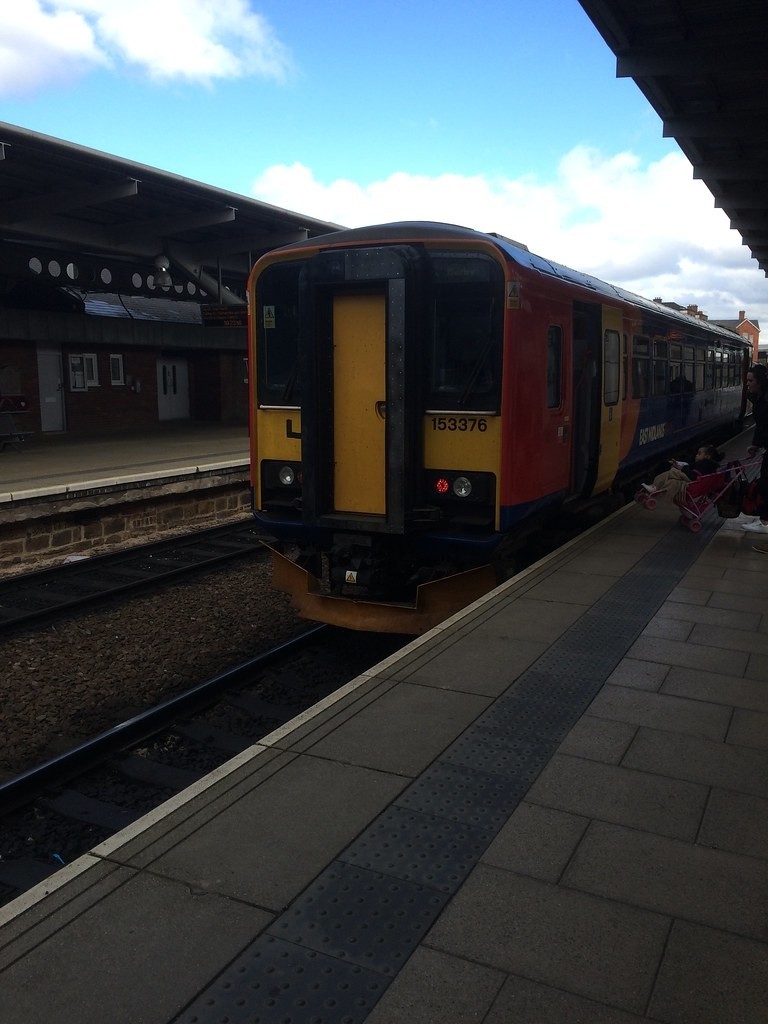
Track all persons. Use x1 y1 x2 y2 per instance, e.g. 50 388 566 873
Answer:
641 445 725 493
745 365 768 554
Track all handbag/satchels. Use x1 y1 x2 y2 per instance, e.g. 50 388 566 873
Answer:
742 478 762 516
717 460 748 518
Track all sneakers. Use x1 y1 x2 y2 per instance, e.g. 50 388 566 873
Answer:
753 542 768 554
742 519 768 534
641 483 657 494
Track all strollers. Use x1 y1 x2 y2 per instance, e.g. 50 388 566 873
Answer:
635 447 763 533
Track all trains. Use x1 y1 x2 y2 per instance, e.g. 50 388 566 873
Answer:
245 220 753 636
